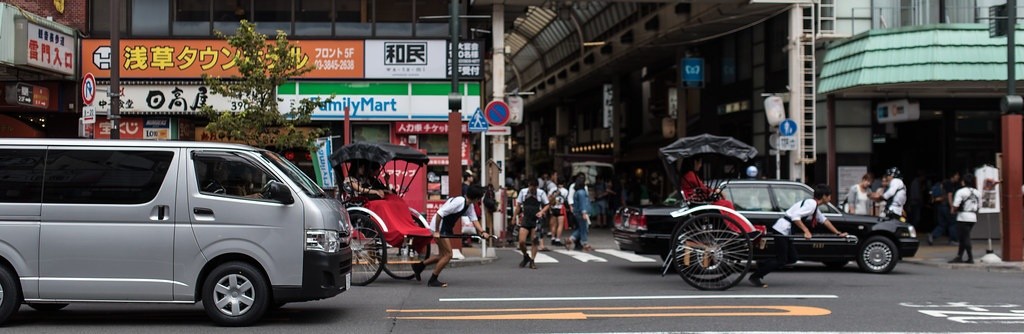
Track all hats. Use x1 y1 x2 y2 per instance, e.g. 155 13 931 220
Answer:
463 169 476 177
887 167 900 178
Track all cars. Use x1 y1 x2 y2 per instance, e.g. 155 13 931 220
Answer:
610 181 920 273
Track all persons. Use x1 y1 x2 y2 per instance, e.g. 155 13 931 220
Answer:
682 155 983 287
222 166 264 201
340 158 660 287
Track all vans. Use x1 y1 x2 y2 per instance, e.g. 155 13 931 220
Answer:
0 138 355 327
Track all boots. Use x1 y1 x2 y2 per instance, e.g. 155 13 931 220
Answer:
411 262 424 281
428 273 448 287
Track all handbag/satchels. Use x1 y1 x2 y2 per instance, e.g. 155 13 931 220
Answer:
484 184 498 212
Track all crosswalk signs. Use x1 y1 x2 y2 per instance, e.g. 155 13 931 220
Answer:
468 109 488 132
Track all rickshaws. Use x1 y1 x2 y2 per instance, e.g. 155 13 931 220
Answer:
328 142 497 286
658 133 858 292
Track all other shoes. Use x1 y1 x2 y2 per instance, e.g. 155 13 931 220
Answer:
551 240 556 245
583 244 594 252
963 259 973 263
555 242 564 246
927 234 933 245
520 254 530 267
564 238 571 249
749 275 767 287
947 257 962 263
529 260 536 269
